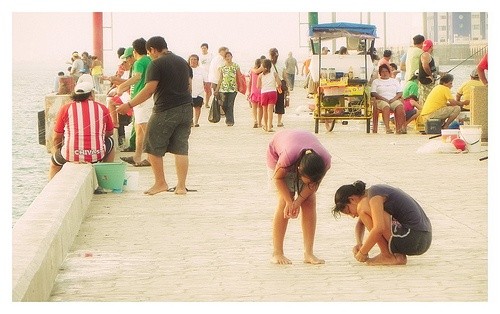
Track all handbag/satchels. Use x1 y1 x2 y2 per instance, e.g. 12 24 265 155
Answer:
207 95 221 123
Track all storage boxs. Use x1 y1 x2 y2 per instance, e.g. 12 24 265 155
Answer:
441 128 459 154
459 124 483 153
208 95 220 123
93 162 126 194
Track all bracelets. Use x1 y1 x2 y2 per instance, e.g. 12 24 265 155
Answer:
358 251 369 258
485 84 489 88
128 102 135 110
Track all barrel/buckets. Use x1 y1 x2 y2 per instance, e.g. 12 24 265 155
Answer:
94 162 127 194
441 128 460 153
459 124 482 153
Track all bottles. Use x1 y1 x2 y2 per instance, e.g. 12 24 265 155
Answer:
319 66 366 82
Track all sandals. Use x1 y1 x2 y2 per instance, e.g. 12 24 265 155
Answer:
120 146 136 153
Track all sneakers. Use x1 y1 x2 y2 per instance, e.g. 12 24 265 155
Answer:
397 127 406 134
386 129 393 133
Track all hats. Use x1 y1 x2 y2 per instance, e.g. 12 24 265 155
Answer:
414 68 420 76
422 40 433 50
74 74 94 94
470 68 479 77
119 48 135 59
413 35 425 45
72 52 79 57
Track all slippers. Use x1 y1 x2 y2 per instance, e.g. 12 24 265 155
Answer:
253 123 286 134
119 156 152 167
145 185 164 194
167 185 196 193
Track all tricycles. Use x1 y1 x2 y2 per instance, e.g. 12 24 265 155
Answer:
306 22 380 134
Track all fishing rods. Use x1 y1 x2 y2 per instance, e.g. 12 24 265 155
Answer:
436 44 487 81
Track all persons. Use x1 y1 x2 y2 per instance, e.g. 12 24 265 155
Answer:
102 48 139 153
456 67 489 112
116 36 193 198
108 88 135 148
332 181 433 267
477 52 489 89
116 38 157 167
420 74 471 131
266 129 333 267
55 34 449 135
47 74 117 183
